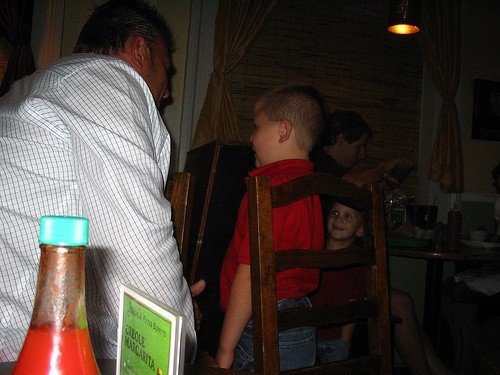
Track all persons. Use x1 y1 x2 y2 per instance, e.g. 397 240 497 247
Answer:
214 86 323 374
308 109 500 375
0 0 206 366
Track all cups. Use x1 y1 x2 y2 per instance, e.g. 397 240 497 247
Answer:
470 230 488 242
430 224 450 251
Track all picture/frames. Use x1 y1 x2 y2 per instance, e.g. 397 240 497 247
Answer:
388 208 406 224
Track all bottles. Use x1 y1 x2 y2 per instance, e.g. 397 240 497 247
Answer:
12 217 101 374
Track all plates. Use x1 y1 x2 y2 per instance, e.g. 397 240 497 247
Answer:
460 240 500 250
387 237 431 249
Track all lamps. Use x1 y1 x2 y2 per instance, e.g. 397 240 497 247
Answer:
386 0 421 35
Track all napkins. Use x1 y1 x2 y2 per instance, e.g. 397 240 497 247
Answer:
453 267 499 296
386 236 426 248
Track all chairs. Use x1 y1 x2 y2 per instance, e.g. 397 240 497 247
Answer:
246 172 393 375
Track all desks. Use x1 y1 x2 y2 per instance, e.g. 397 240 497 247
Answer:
386 250 500 356
